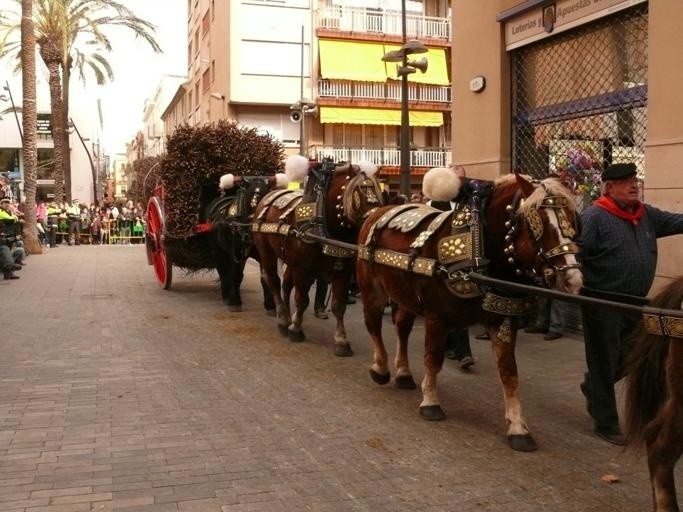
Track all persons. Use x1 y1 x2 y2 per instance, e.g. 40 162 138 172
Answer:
33 196 148 246
0 172 26 279
571 162 682 448
524 294 570 342
444 327 474 370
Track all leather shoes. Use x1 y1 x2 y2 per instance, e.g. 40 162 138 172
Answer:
475 332 491 342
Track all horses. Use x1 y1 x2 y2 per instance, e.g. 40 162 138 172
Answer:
356 167 584 452
620 273 683 512
203 163 385 357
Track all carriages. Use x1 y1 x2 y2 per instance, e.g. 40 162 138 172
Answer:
139 119 682 512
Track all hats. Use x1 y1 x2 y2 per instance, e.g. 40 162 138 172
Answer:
1 197 11 203
601 163 638 181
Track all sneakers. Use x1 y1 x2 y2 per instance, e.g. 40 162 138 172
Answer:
460 357 476 370
594 421 626 446
544 331 563 341
16 260 27 266
4 274 20 280
5 264 22 272
525 324 547 334
447 350 459 361
580 382 596 418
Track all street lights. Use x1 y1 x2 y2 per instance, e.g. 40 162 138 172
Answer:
380 39 429 197
290 97 318 157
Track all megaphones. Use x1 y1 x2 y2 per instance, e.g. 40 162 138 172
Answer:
396 64 416 77
406 56 427 73
290 111 302 123
304 106 318 119
